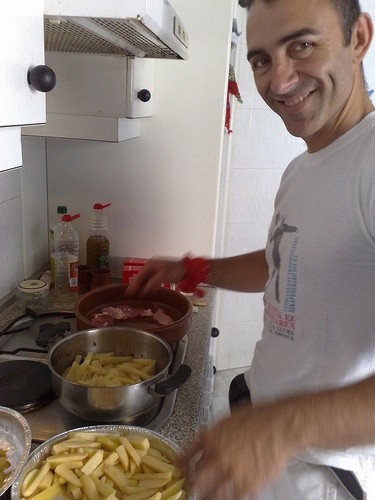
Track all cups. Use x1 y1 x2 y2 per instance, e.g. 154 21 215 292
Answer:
78 265 112 294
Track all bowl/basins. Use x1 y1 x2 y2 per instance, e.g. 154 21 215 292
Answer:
11 425 197 500
0 405 32 497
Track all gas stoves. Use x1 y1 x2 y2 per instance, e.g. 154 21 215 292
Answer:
0 308 190 444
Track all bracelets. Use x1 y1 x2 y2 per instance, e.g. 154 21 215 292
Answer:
179 255 210 285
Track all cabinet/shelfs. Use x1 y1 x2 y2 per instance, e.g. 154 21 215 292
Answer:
0 0 244 259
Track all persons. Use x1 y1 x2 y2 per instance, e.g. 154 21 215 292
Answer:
125 0 375 500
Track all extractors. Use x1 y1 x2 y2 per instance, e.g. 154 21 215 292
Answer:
43 0 191 61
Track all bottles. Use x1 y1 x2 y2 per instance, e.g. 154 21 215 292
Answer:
86 201 111 270
49 205 68 287
55 213 80 296
16 280 49 311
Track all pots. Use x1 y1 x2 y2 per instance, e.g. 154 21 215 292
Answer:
36 321 193 423
74 283 194 346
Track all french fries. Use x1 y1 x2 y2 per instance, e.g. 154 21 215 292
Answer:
60 352 158 388
0 449 11 489
21 432 189 500
